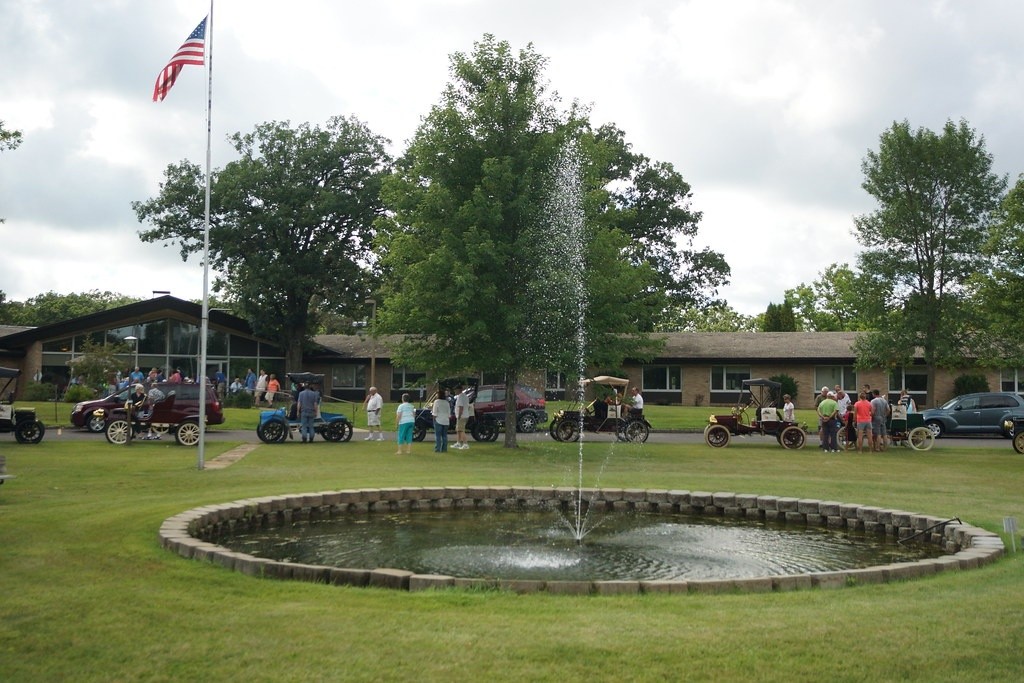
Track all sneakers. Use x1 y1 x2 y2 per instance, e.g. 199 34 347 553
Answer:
364 436 375 441
458 444 470 450
449 442 461 450
377 436 385 441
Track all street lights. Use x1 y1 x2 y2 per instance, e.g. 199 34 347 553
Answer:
365 298 376 389
195 306 232 382
122 335 138 447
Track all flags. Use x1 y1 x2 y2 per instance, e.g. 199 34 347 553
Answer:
152 14 207 103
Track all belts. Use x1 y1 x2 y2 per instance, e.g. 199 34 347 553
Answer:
368 410 376 412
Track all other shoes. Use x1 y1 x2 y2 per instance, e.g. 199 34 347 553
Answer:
138 433 161 440
822 448 828 453
856 447 887 454
831 448 842 453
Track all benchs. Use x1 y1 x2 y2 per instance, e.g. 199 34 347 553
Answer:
756 400 778 421
0 391 15 405
151 390 175 418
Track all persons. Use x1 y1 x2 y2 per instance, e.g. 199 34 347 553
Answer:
255 368 268 406
206 368 225 398
431 384 469 452
125 383 165 439
147 367 164 382
110 366 144 395
170 368 185 382
297 381 318 443
287 382 322 419
364 387 385 441
245 368 257 395
782 394 794 421
814 385 851 452
230 376 243 392
844 405 858 451
898 390 916 411
395 393 415 455
265 374 280 407
853 384 890 454
621 387 643 418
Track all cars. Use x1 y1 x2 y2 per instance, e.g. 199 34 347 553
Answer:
915 390 1024 439
441 385 550 433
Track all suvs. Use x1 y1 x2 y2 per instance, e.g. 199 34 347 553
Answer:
70 382 227 434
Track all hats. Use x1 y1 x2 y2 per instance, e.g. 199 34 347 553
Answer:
453 384 463 390
